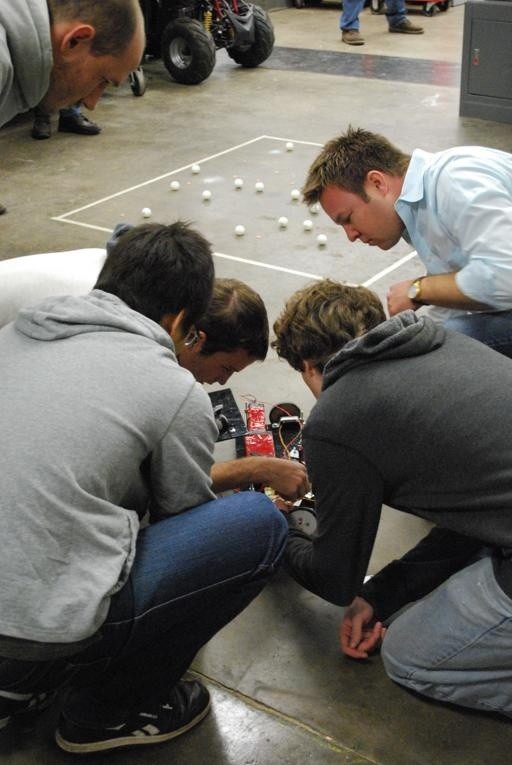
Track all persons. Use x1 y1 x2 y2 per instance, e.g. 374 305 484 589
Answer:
1 0 144 130
2 219 289 753
338 1 425 45
31 105 103 141
272 278 512 715
301 122 512 359
1 247 312 502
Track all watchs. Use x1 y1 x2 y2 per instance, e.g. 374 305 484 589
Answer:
406 276 429 306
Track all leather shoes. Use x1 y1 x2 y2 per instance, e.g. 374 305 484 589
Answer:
32 113 51 137
339 29 365 44
59 113 102 132
390 19 423 33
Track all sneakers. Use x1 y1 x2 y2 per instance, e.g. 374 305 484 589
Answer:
52 676 212 754
0 672 72 736
